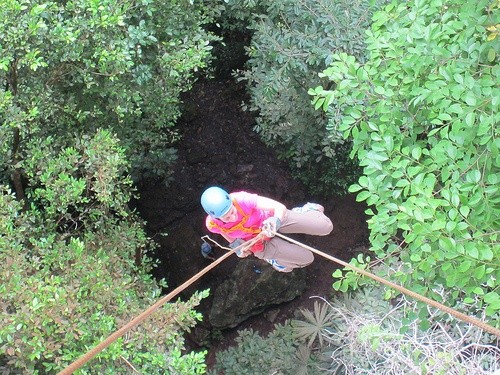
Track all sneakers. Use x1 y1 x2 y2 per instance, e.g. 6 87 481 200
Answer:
292 202 324 215
264 259 293 273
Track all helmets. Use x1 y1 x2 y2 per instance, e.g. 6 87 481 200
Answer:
201 186 233 219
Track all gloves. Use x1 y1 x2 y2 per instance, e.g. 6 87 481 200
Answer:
228 238 252 258
261 216 282 237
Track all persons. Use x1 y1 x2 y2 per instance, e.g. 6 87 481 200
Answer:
201 187 333 273
201 243 219 261
